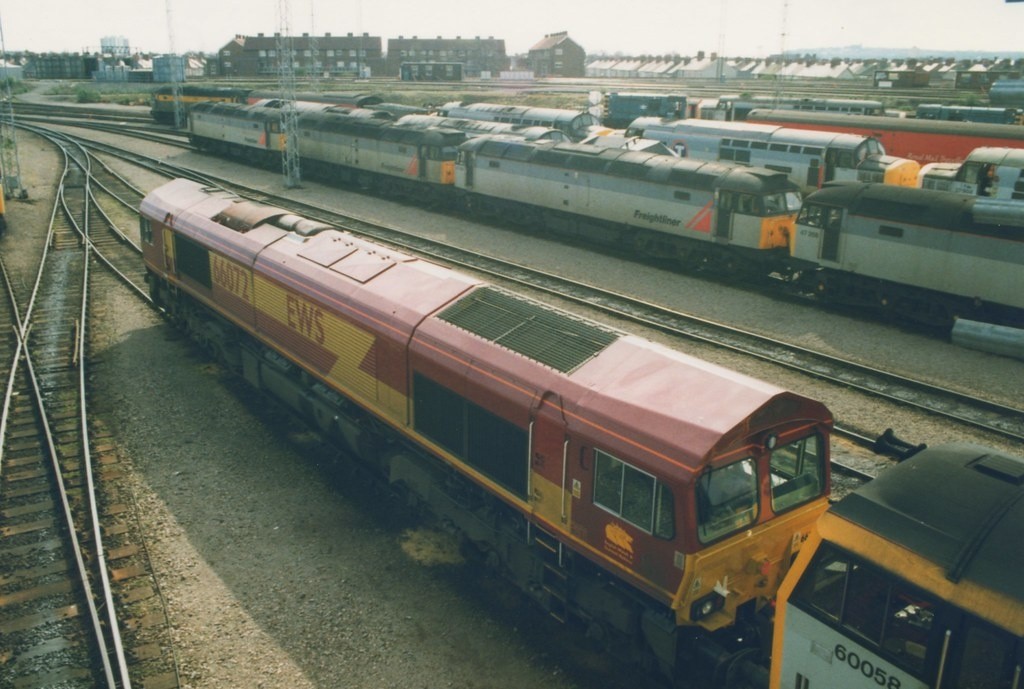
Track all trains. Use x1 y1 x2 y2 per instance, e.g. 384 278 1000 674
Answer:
152 75 1024 341
140 175 1024 688
